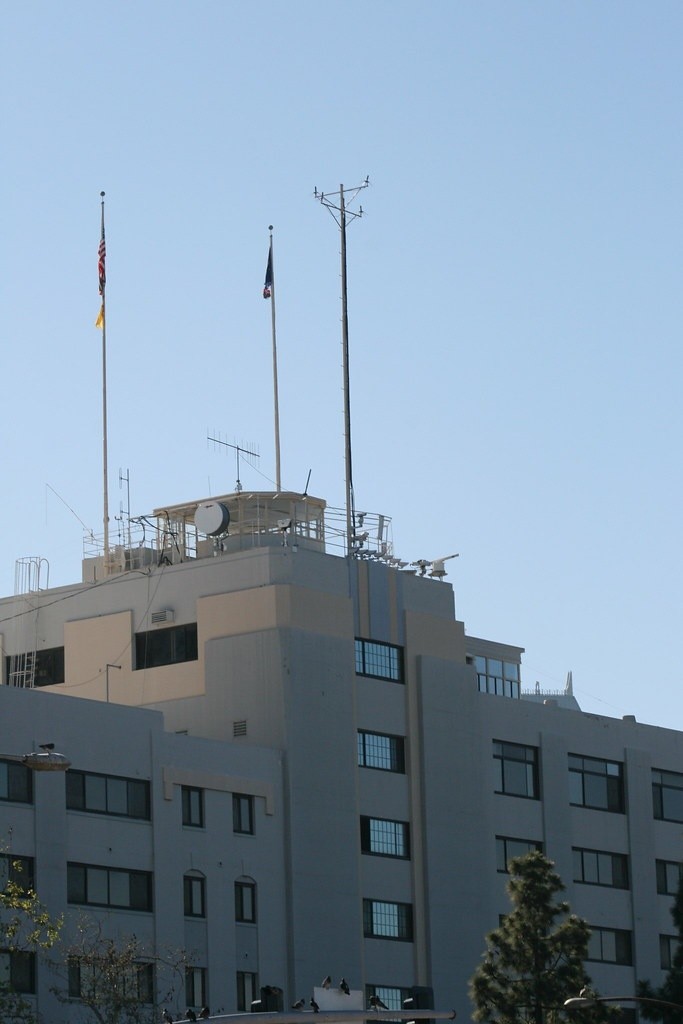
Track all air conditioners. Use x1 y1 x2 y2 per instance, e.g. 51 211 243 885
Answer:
151 610 174 625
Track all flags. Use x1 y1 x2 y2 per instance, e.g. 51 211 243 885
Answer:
95 303 104 329
263 247 272 298
97 229 107 296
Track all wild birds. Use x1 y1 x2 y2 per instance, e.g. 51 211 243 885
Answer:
339 978 350 995
292 998 305 1011
321 976 332 990
186 1008 196 1023
162 1008 174 1024
368 995 389 1011
199 1004 210 1020
310 997 319 1013
579 985 588 998
